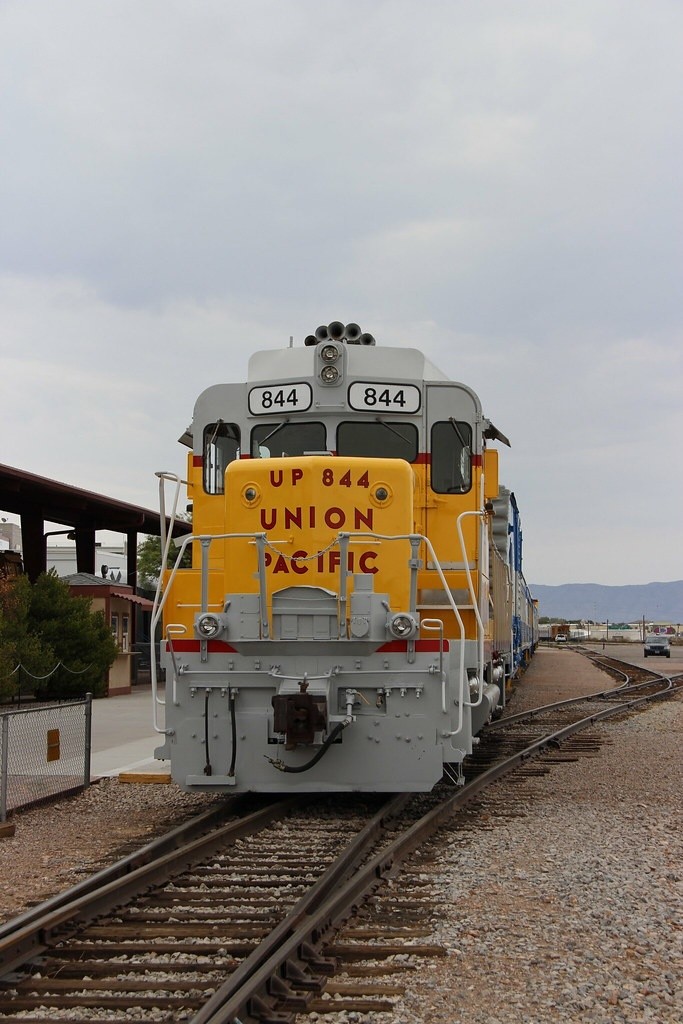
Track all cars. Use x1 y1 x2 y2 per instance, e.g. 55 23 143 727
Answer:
554 634 566 641
642 636 673 658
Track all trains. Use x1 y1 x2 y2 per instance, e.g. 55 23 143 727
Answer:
144 320 542 797
